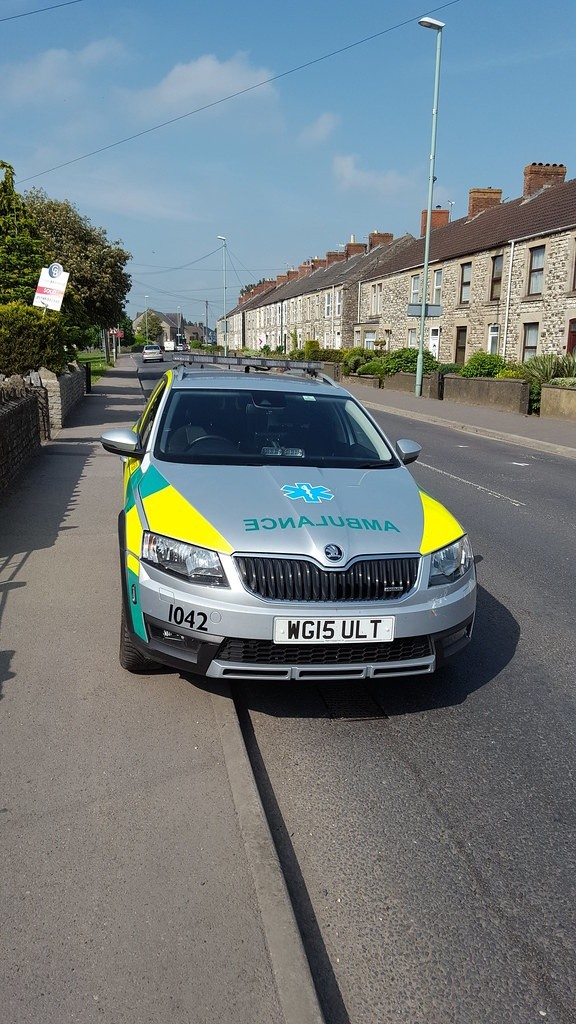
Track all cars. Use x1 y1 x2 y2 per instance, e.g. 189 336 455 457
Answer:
175 346 184 352
142 345 164 363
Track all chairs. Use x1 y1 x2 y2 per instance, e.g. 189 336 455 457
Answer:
269 406 335 455
168 405 231 450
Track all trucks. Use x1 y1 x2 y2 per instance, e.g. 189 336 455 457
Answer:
163 341 174 352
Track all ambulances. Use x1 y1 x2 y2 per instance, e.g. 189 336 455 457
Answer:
100 352 477 681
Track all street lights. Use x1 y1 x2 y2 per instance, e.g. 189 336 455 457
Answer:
145 295 149 345
413 16 446 398
217 235 228 356
